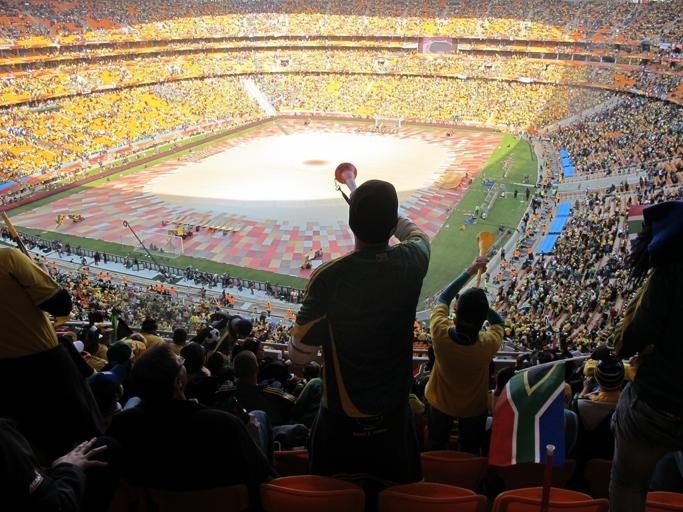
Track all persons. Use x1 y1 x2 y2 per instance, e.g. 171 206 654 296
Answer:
460 122 683 230
0 0 246 228
245 0 682 124
286 179 430 489
0 229 683 511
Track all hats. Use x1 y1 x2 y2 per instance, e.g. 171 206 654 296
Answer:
89 360 130 399
454 287 489 319
594 359 625 379
348 179 399 228
